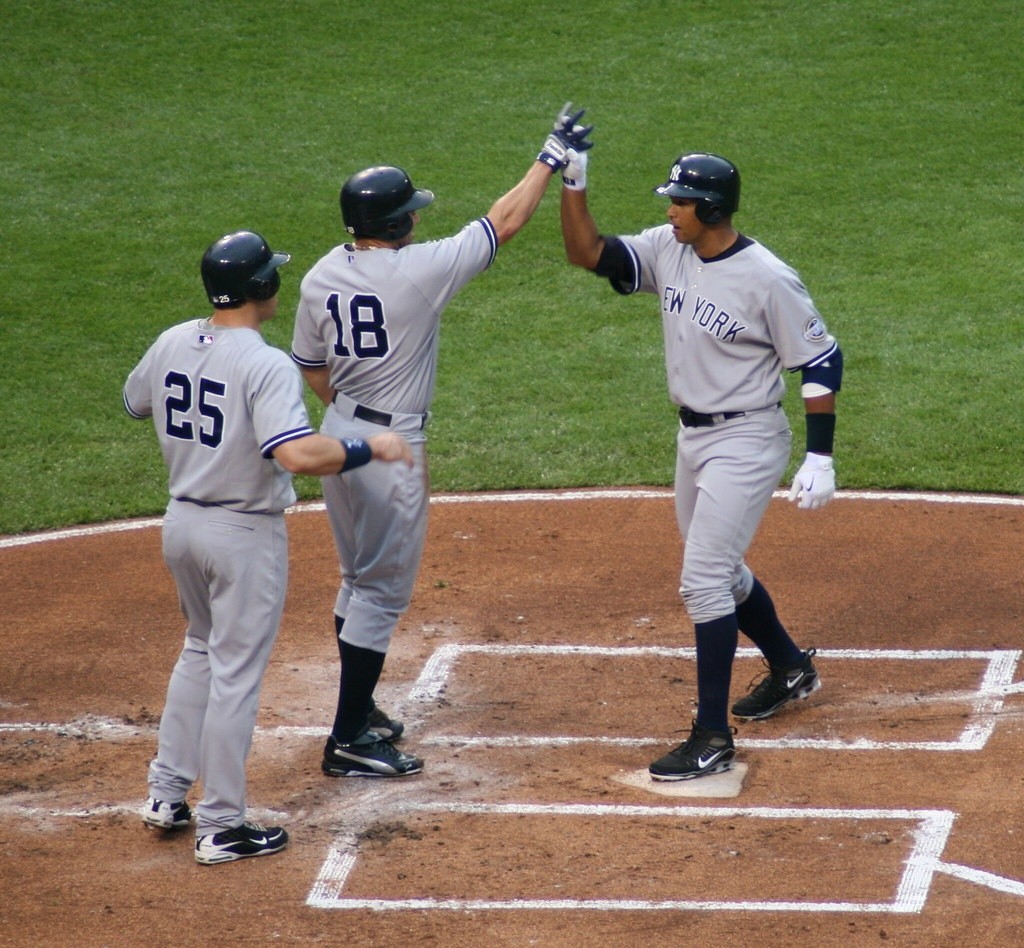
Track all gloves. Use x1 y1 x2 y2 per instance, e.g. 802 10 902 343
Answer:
536 102 594 173
788 452 836 510
554 116 587 191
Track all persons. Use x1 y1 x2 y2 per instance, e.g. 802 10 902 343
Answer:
289 100 595 778
123 229 417 864
561 121 845 782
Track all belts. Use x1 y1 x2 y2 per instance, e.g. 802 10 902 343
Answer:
678 400 782 428
331 391 427 430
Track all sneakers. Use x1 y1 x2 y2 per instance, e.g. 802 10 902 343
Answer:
649 717 738 782
731 646 822 721
322 723 424 778
367 698 404 742
141 796 191 829
194 820 288 865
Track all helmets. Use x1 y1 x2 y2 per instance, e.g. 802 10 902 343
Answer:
340 166 434 239
201 230 291 304
652 153 741 223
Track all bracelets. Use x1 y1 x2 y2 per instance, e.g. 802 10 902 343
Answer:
338 436 373 476
804 412 836 454
